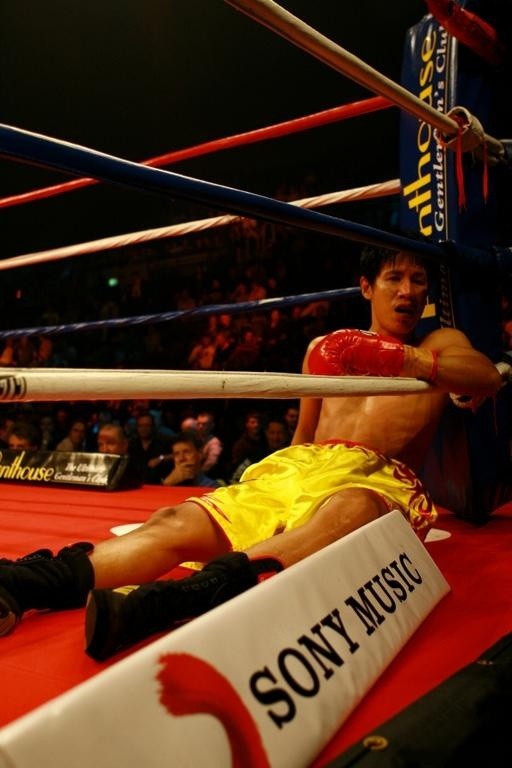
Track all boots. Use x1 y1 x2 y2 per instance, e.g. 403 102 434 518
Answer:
1 540 96 619
84 551 259 665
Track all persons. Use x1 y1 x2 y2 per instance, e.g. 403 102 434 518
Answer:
1 259 332 487
0 227 502 662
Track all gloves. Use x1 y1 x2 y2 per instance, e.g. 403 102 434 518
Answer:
307 328 440 384
